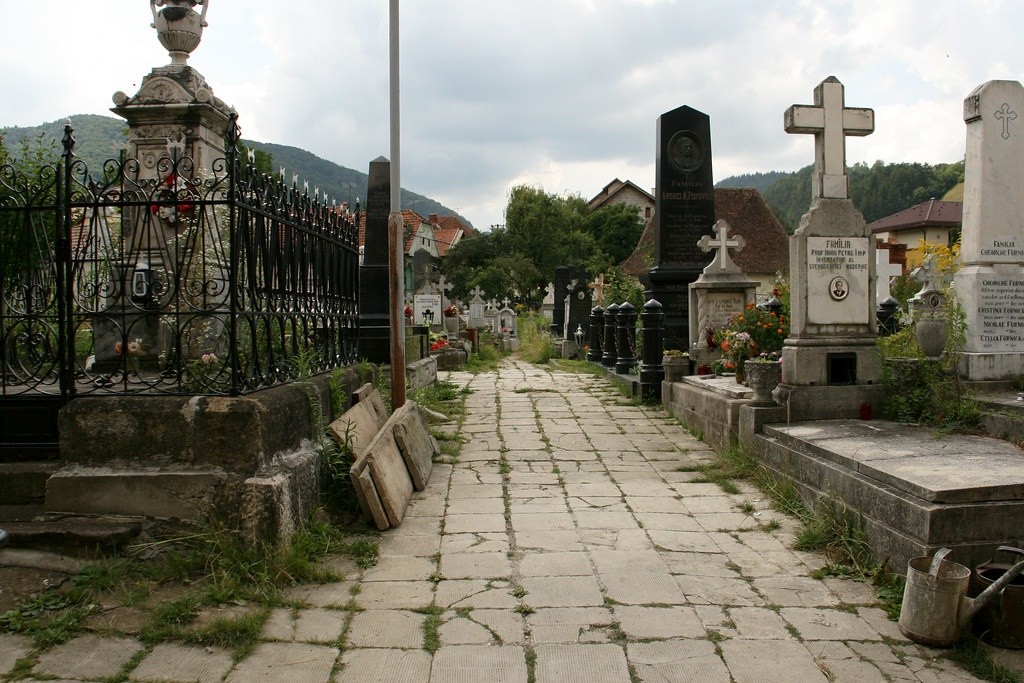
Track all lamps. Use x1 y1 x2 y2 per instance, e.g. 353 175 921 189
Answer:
574 323 585 351
421 309 434 326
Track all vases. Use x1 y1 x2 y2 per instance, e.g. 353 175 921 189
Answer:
916 318 949 357
744 361 781 406
446 317 458 339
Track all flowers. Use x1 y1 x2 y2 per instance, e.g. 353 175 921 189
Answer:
749 352 783 364
443 303 459 317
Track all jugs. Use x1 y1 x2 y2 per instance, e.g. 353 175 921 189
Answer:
969 545 1024 650
897 547 1024 646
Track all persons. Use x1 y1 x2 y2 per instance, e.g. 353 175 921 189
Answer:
833 281 846 297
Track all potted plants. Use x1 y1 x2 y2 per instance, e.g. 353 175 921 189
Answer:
662 350 690 382
